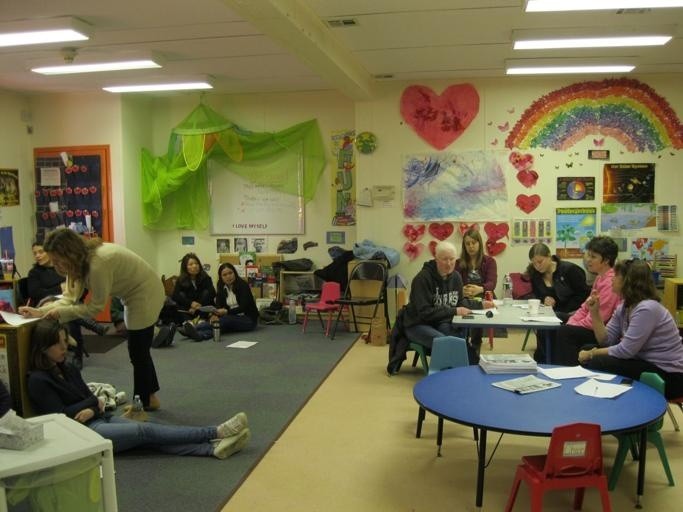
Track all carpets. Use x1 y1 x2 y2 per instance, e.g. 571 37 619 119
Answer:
78 316 363 512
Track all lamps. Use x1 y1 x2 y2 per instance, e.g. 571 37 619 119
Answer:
1 15 93 47
100 75 215 94
25 52 164 77
502 1 682 76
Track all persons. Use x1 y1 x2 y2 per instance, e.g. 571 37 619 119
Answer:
77 287 178 347
26 319 251 460
176 263 259 342
27 241 83 370
578 258 683 400
159 253 216 328
403 241 479 365
18 227 166 411
534 237 619 367
527 243 586 363
455 230 497 365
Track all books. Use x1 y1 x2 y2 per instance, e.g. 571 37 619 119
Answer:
479 353 538 375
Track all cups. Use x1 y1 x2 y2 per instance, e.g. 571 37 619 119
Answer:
0 260 13 280
528 299 541 316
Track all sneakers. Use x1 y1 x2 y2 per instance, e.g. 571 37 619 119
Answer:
121 397 160 423
212 413 250 459
153 322 202 347
98 326 109 334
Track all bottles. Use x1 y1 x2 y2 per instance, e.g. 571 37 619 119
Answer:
212 320 221 341
503 272 513 306
131 395 143 421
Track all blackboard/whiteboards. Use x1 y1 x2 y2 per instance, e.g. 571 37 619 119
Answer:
206 140 305 236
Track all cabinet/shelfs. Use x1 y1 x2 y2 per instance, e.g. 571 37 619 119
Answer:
217 252 316 304
347 260 388 333
1 412 116 512
1 315 71 420
660 277 683 330
0 275 27 314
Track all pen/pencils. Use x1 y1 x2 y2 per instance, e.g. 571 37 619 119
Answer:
23 297 31 315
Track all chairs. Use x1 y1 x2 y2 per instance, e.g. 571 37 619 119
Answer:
505 421 612 511
666 398 683 432
390 271 533 446
301 282 346 338
608 370 675 492
331 261 393 344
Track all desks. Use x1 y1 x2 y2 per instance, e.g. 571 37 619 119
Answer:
451 297 563 363
412 365 669 509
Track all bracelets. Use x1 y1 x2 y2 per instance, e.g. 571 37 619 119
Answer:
589 349 594 362
